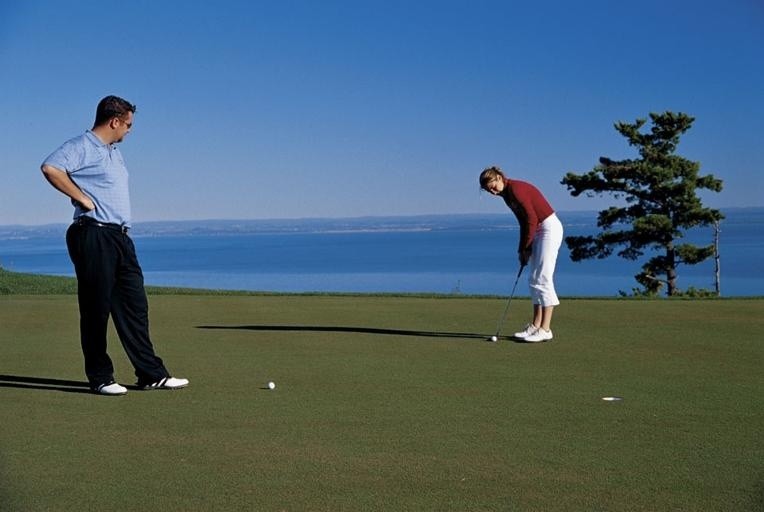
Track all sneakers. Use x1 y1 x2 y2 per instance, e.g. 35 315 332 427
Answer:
513 322 537 340
90 379 127 395
138 375 191 390
523 328 553 343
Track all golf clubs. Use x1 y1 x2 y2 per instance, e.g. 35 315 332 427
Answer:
487 265 524 340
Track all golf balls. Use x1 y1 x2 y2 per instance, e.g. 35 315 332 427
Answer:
269 382 275 389
492 336 497 341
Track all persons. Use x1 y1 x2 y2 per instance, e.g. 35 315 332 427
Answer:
480 163 566 343
40 95 188 394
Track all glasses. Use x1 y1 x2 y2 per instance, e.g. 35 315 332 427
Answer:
119 118 133 129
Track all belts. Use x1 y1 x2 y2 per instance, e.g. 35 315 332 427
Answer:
73 216 130 235
